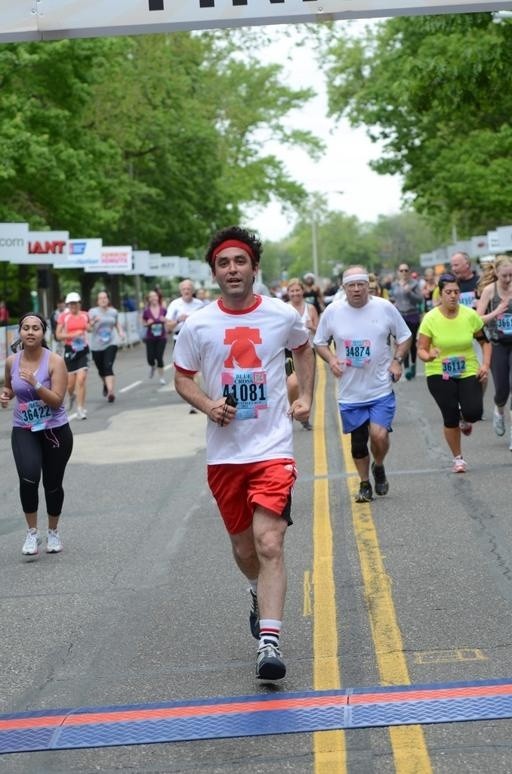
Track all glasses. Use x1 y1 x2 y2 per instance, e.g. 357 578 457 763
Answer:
398 269 409 273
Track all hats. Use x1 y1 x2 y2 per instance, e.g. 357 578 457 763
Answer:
65 291 81 303
368 282 378 291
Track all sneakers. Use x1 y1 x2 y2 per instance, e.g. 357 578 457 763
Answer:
21 528 42 556
45 528 63 553
108 395 115 402
77 410 87 420
255 638 287 680
148 367 155 378
371 461 389 496
158 378 166 385
452 456 466 473
65 393 76 411
492 405 506 436
189 406 198 414
509 426 512 451
459 409 472 436
355 481 372 502
249 588 262 640
103 389 108 397
410 364 416 377
404 368 412 380
301 421 313 431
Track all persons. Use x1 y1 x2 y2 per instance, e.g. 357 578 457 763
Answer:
165 280 203 415
0 312 74 555
172 227 317 682
313 265 413 504
51 280 208 420
391 252 511 471
275 276 318 431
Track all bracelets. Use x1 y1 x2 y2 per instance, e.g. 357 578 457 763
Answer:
393 357 405 363
33 382 42 390
